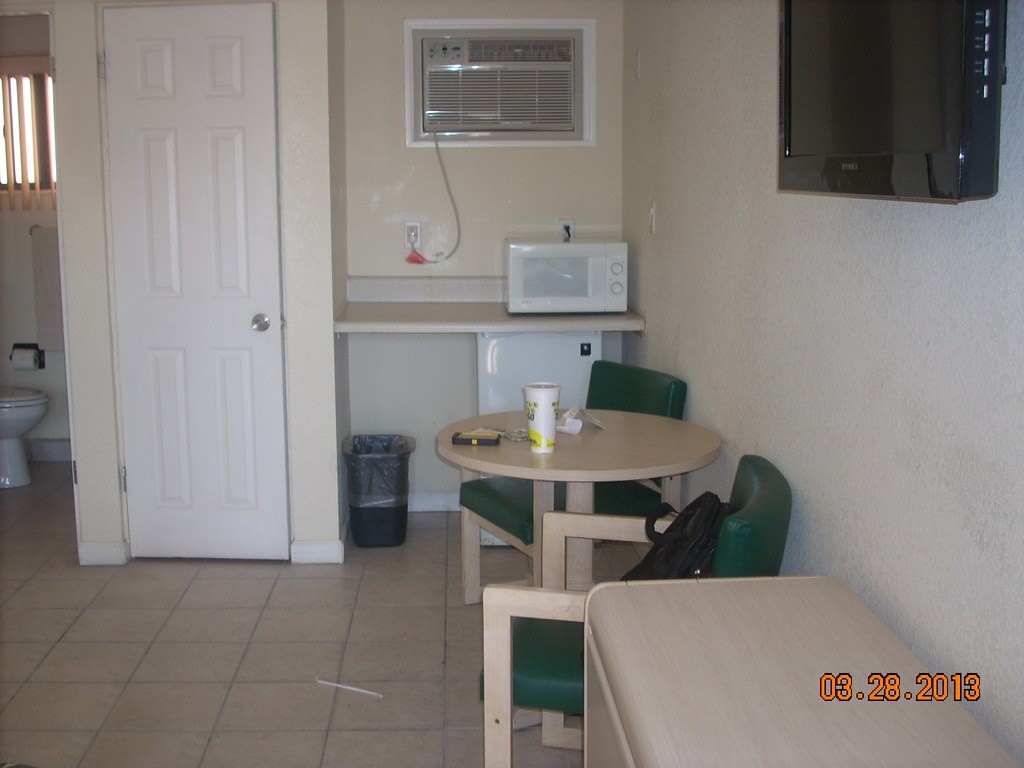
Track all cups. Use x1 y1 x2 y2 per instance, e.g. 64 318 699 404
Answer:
525 382 561 454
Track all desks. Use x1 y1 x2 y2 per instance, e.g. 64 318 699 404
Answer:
338 303 643 548
582 575 1022 768
435 410 723 727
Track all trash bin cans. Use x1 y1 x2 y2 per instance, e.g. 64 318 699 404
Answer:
340 432 417 549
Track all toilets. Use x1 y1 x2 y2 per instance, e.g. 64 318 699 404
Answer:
1 384 48 491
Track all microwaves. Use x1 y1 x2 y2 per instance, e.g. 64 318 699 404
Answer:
504 238 627 313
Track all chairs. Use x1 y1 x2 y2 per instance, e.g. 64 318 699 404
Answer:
464 359 684 607
483 454 793 768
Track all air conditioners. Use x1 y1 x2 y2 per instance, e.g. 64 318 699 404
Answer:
423 38 573 131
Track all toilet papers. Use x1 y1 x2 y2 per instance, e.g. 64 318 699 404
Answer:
11 345 38 372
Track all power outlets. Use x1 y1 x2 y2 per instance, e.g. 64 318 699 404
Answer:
404 222 420 249
